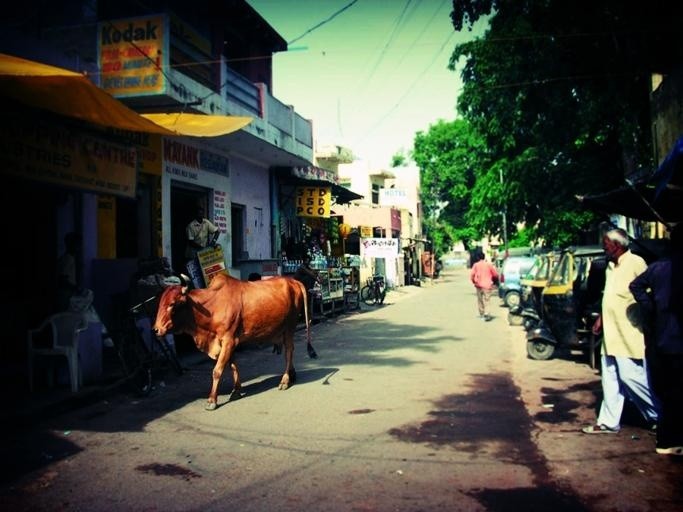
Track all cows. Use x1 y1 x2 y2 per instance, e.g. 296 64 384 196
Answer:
150 273 316 411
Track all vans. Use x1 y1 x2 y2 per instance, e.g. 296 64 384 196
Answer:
490 244 555 311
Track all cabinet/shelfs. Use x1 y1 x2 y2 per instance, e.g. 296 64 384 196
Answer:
281 260 360 299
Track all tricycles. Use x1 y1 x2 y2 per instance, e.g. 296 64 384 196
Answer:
520 256 559 329
507 251 563 326
525 245 606 360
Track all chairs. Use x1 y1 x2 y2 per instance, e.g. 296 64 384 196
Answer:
566 259 605 329
25 310 88 391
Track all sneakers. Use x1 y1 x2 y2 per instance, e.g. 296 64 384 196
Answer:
655 442 682 455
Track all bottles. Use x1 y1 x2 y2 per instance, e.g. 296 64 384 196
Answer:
283 259 303 272
312 255 327 270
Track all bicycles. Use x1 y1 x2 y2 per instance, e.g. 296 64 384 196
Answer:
115 273 182 393
359 272 386 306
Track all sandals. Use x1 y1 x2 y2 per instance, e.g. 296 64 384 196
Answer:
582 425 618 434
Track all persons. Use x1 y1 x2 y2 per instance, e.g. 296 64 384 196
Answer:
628 222 682 456
185 207 223 273
586 228 663 435
293 255 322 317
472 252 499 322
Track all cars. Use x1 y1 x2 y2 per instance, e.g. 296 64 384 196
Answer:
443 255 467 267
